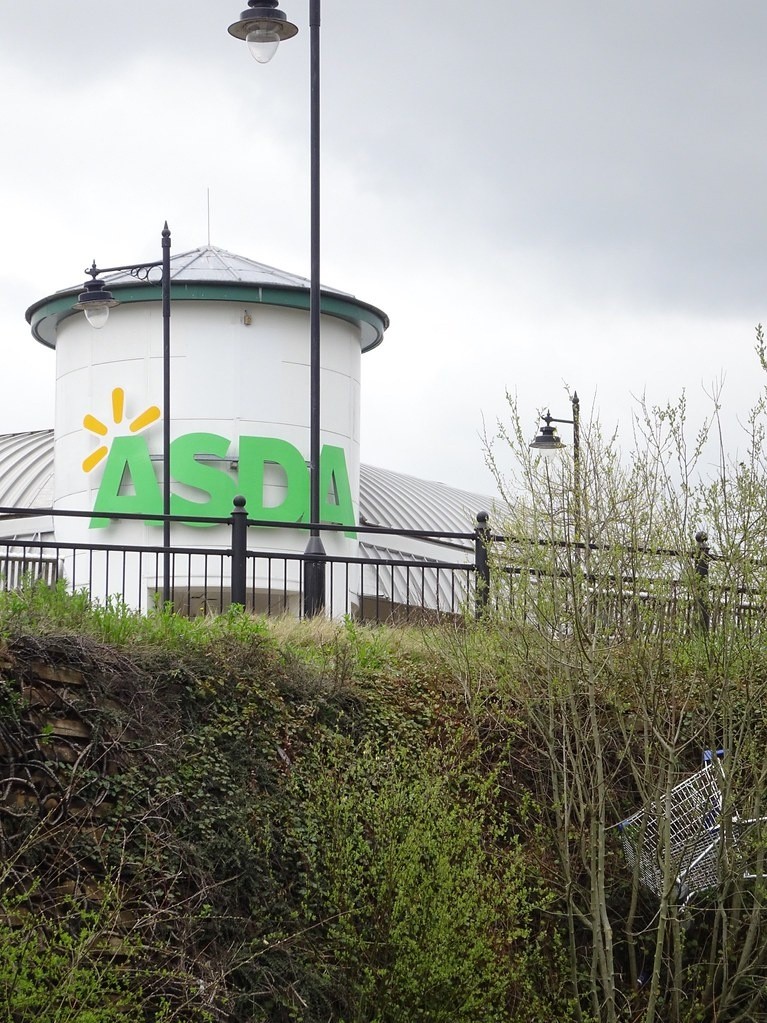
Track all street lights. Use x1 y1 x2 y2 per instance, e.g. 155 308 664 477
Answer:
527 388 588 651
226 1 332 623
70 218 172 617
